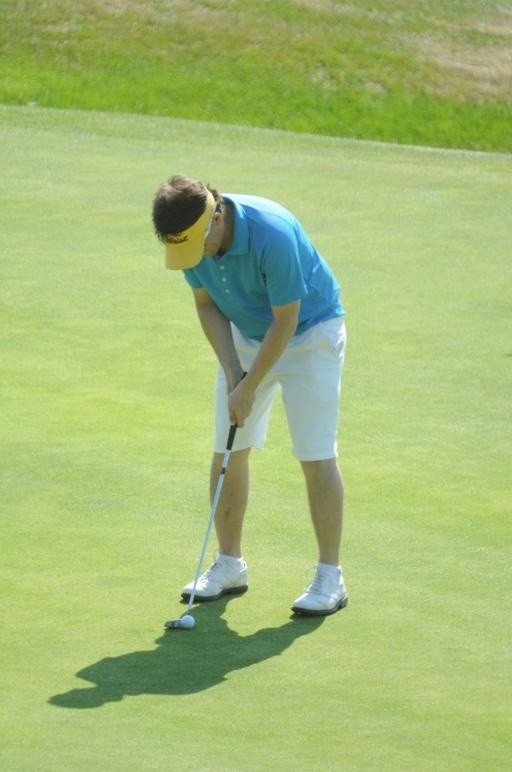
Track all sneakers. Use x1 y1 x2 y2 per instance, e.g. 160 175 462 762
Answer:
181 557 249 602
290 569 348 616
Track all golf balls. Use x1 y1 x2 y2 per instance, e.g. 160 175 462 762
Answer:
181 615 194 627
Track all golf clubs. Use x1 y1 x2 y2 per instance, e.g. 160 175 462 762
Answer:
163 371 251 627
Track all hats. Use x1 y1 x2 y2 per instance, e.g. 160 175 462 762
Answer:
159 182 217 269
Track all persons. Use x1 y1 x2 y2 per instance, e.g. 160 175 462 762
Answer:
150 176 347 616
